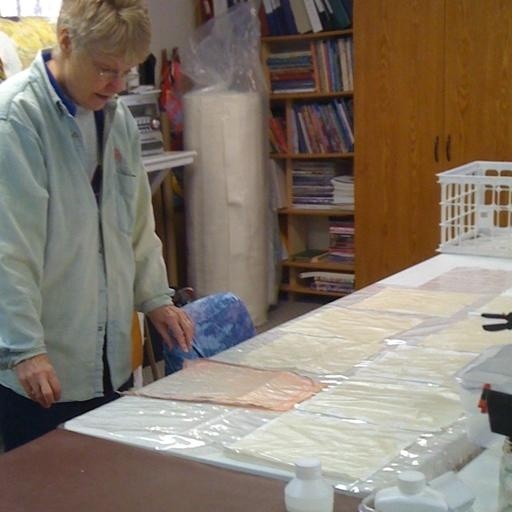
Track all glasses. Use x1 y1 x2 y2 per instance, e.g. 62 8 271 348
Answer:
93 65 138 84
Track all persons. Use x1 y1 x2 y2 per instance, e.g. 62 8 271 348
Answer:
0 0 193 451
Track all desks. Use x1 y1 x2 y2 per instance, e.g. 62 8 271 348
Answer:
0 238 512 512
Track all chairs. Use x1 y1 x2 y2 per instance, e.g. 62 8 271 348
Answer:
162 292 255 375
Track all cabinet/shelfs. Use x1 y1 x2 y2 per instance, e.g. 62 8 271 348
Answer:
353 1 512 293
258 1 354 305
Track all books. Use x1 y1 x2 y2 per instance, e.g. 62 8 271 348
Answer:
267 115 288 154
292 217 355 263
269 159 288 209
267 38 354 93
300 271 356 293
291 157 355 210
292 98 354 153
